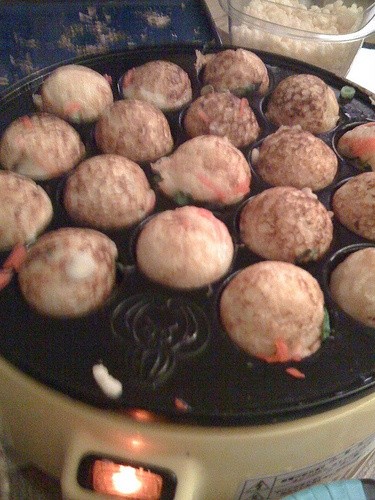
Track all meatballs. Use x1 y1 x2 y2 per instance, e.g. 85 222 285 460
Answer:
0 46 375 362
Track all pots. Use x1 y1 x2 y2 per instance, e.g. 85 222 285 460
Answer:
0 41 374 500
